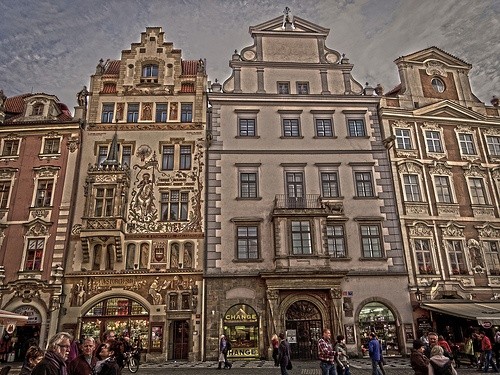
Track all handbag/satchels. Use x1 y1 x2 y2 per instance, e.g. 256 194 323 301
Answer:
218 351 225 363
286 360 292 370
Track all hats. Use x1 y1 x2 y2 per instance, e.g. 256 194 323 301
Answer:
413 340 424 349
423 330 426 333
494 327 498 330
278 333 284 337
372 332 376 337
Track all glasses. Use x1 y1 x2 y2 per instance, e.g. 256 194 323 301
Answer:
103 344 107 348
57 344 71 349
84 345 93 348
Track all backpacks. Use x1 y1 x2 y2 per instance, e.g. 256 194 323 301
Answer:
226 341 231 350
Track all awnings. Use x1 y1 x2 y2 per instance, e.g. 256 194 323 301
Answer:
421 302 500 326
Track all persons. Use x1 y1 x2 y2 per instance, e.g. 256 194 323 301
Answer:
317 329 342 375
216 334 232 369
463 329 500 372
0 335 19 365
278 333 290 375
333 335 351 375
369 332 387 375
411 330 457 375
20 332 136 375
271 333 279 367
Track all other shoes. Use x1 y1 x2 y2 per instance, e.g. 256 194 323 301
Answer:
216 367 221 369
229 364 232 370
